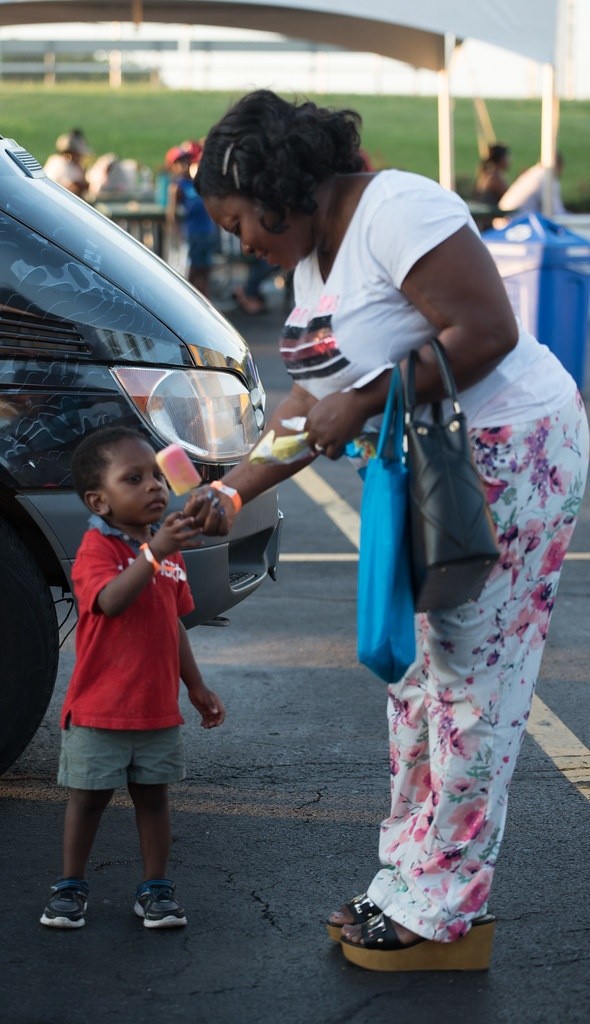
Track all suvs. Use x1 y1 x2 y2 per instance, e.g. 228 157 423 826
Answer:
0 135 284 775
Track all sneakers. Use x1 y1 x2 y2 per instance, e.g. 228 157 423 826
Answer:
41 876 89 927
133 878 187 928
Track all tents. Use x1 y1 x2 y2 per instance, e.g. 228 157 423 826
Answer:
0 0 559 218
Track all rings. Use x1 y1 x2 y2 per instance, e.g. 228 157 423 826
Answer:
314 442 324 452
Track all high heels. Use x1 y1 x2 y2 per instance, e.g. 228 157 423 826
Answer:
326 892 382 945
340 911 495 971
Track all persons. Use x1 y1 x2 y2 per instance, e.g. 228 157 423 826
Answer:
229 259 295 316
39 426 226 927
471 145 510 231
492 153 565 231
165 146 216 294
193 88 590 970
41 131 89 196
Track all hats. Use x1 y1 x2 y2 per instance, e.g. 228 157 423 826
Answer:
56 129 92 156
165 146 194 169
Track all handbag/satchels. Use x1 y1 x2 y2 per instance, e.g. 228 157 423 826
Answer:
356 361 411 682
406 338 500 612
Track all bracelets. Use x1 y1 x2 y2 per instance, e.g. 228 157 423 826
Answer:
139 543 174 574
210 481 242 513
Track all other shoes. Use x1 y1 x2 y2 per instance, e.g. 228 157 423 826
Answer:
232 288 264 313
